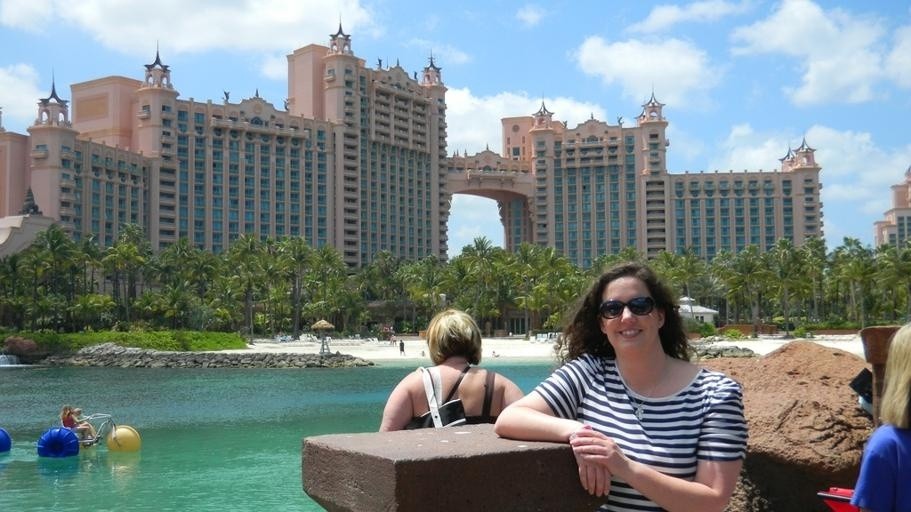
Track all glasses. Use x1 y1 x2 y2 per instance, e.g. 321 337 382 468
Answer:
599 297 656 320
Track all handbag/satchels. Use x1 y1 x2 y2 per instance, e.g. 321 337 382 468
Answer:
418 399 466 429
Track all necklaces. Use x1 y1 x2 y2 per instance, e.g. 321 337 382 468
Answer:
614 357 673 421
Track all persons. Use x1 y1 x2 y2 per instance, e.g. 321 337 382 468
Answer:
392 334 397 346
850 320 911 512
63 405 96 440
71 407 98 436
496 257 752 511
377 308 525 432
399 339 405 357
